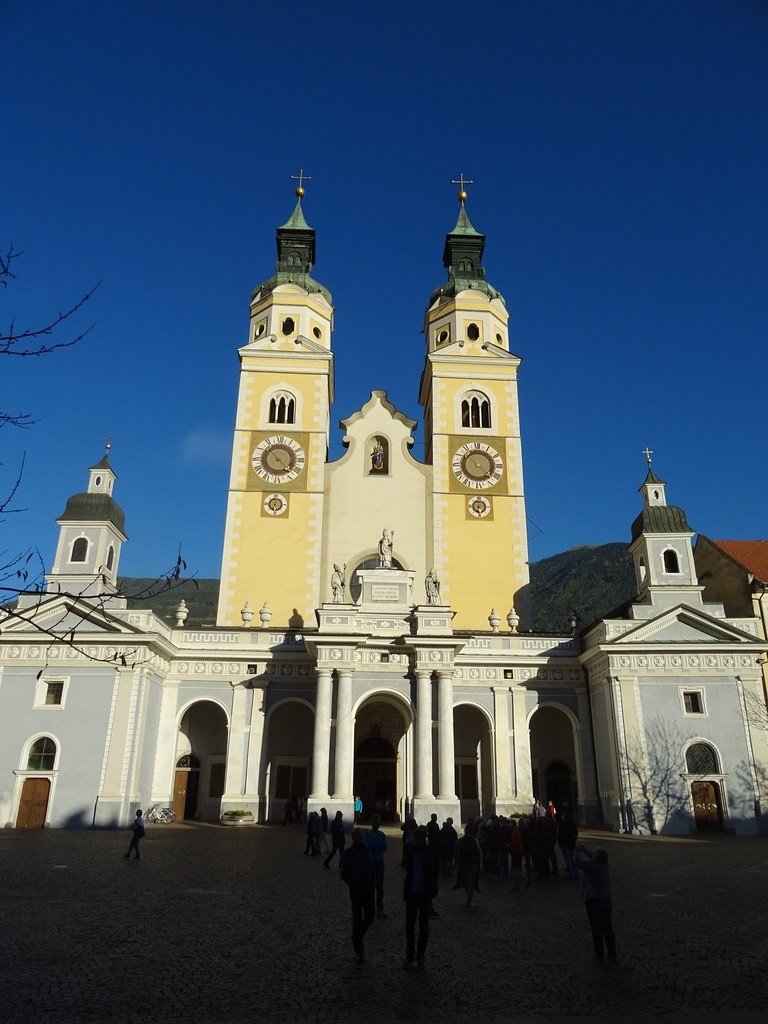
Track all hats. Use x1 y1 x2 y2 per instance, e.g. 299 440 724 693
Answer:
595 846 609 862
336 810 343 815
352 827 363 835
320 807 327 813
371 815 382 825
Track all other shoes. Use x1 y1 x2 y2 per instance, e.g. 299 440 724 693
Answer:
452 881 460 890
323 861 331 869
429 910 441 919
356 950 365 961
441 871 446 875
282 822 286 825
289 821 293 824
525 880 531 889
403 956 415 967
310 853 316 856
323 850 330 855
295 819 302 823
565 875 571 879
417 957 425 968
564 867 568 870
509 887 520 892
377 911 387 918
550 868 559 874
302 851 308 856
315 851 321 855
448 872 454 876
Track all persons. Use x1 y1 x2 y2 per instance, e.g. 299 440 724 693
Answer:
425 570 441 604
332 563 344 603
378 529 392 567
284 793 639 973
123 809 145 861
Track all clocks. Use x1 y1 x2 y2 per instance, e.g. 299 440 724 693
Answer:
454 442 504 489
252 436 306 484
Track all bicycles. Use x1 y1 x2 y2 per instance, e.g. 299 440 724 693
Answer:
143 802 178 824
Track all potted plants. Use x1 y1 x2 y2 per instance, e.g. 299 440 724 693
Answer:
221 810 254 825
510 812 528 825
